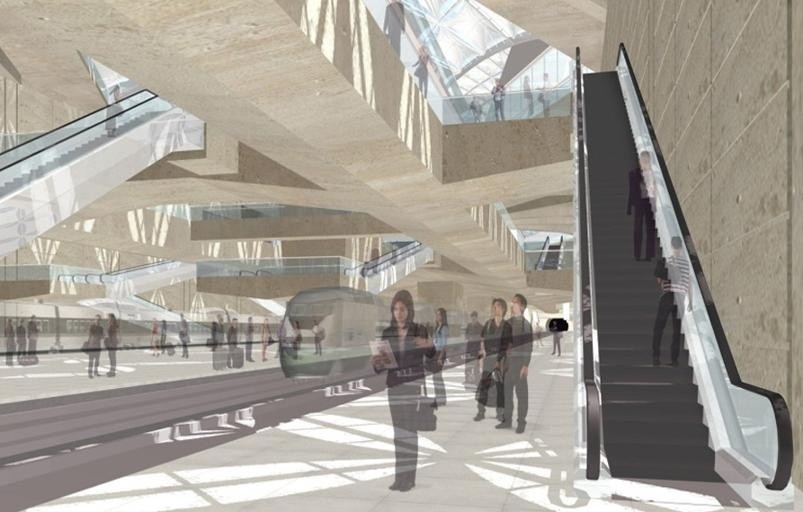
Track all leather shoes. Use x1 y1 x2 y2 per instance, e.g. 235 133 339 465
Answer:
653 360 660 365
389 480 400 490
474 411 525 433
671 360 678 366
88 371 115 377
399 480 414 491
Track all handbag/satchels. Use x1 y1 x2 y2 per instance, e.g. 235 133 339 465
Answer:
417 397 436 431
474 367 504 407
654 256 672 281
81 341 89 354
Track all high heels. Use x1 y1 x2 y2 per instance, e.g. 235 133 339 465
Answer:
434 398 445 405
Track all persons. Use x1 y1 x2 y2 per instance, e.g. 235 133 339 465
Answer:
627 151 659 261
414 46 428 97
550 322 561 356
89 314 104 378
384 0 405 58
151 313 324 367
469 72 553 122
4 314 38 366
536 323 543 346
652 235 694 366
105 313 117 377
369 290 533 492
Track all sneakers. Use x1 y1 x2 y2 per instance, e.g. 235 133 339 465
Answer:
248 359 255 363
263 358 267 361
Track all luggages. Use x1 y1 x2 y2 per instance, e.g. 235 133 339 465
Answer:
166 342 174 355
231 347 243 368
212 350 228 370
